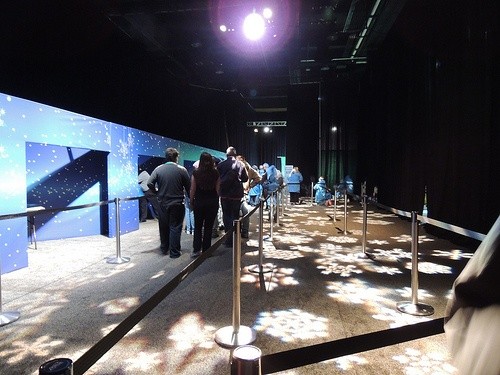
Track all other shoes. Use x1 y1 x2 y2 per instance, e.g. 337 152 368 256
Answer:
202 243 211 252
170 250 183 257
222 240 233 248
186 229 194 235
193 246 201 252
211 230 220 238
161 250 169 254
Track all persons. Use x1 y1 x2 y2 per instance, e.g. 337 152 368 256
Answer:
314 175 353 208
146 146 192 258
185 155 305 234
137 167 160 224
218 146 249 236
189 152 221 256
